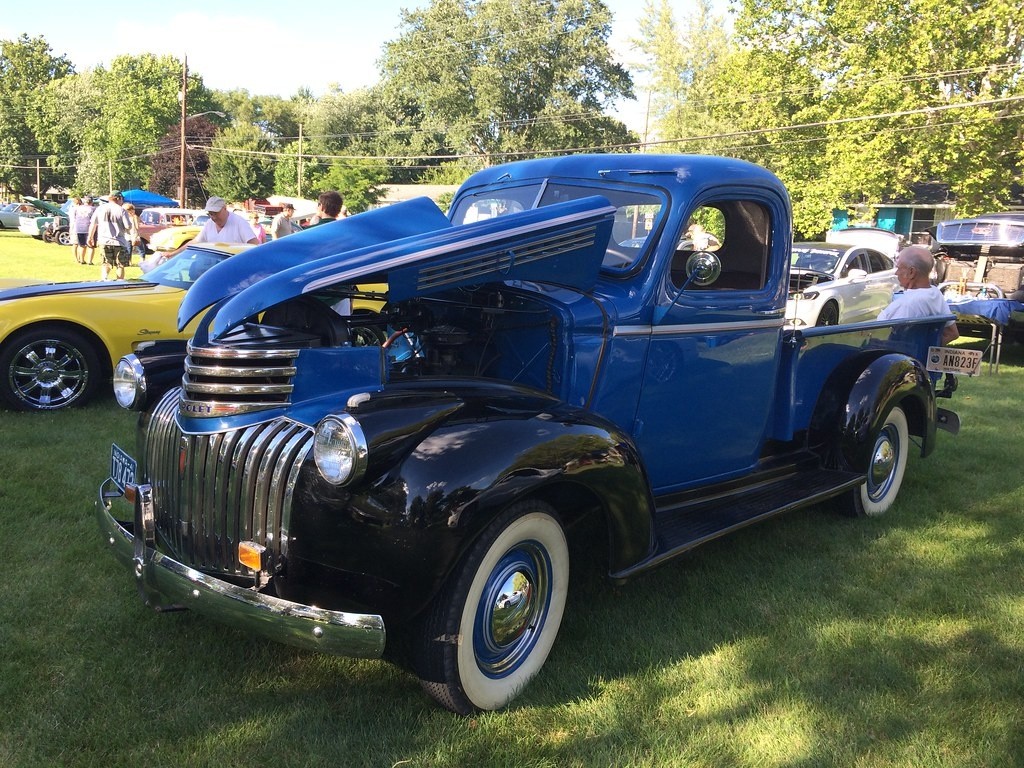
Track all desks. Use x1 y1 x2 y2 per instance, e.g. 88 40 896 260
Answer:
892 291 1024 326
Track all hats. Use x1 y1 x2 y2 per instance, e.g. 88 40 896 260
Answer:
284 204 296 210
109 190 124 197
250 213 259 217
205 197 225 212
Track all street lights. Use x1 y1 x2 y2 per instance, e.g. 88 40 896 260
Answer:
179 110 227 220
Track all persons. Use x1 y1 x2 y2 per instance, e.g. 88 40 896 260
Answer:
689 224 718 246
122 203 145 266
164 197 259 258
87 190 132 280
878 247 960 347
303 190 354 344
69 197 82 263
75 198 97 265
271 203 296 239
250 213 267 244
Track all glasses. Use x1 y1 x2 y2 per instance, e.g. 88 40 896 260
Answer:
252 217 259 219
206 212 219 216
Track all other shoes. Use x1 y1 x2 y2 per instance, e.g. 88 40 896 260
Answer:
89 263 94 265
81 261 86 264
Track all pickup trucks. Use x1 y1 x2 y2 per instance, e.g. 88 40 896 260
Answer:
94 151 986 718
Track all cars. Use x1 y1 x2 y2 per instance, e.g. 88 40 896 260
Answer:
0 198 1024 412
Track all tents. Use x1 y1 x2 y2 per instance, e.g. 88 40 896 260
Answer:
121 188 178 206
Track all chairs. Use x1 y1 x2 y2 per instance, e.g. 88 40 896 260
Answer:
937 281 1005 376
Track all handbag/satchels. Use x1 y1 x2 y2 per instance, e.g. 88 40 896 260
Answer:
131 231 141 246
